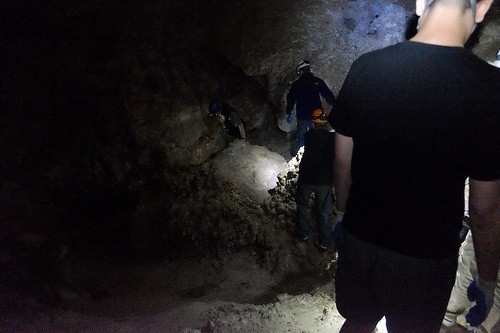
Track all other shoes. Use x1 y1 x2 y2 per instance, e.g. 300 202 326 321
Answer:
295 229 310 240
319 238 330 249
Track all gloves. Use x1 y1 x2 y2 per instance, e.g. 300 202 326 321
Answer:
459 216 473 241
218 114 226 122
466 279 496 327
286 115 292 122
332 207 346 241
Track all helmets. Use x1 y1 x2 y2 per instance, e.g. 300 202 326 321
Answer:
209 102 221 113
297 59 310 74
312 109 328 123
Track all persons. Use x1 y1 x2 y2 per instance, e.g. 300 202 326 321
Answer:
330 0 500 333
294 110 337 251
286 60 338 149
